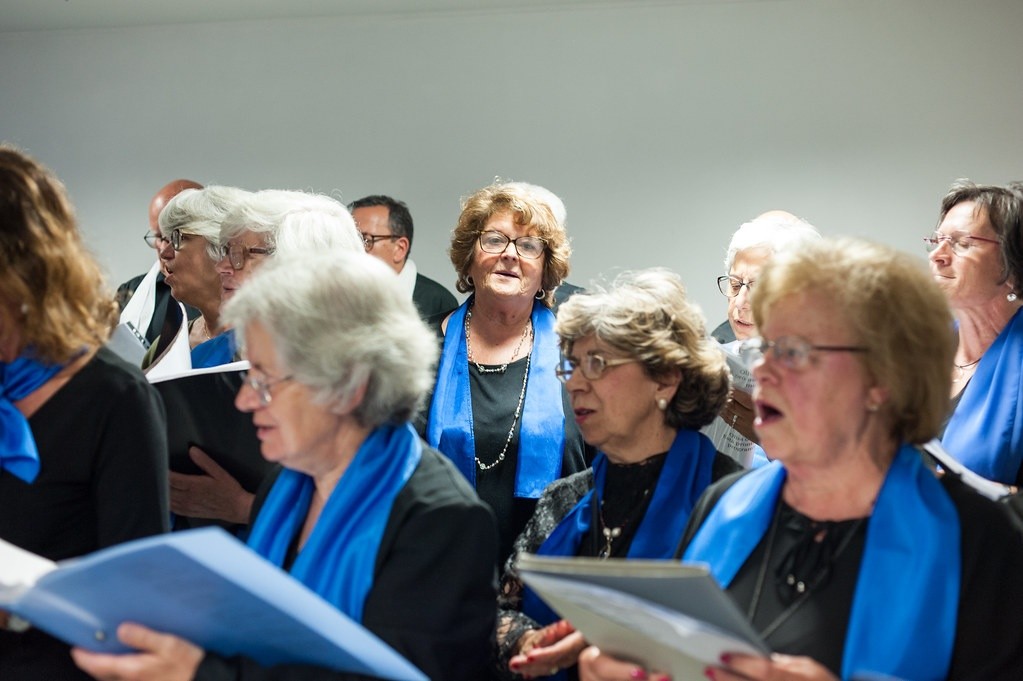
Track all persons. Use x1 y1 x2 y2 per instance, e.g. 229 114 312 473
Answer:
0 143 1023 681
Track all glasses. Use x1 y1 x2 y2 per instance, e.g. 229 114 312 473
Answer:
144 230 169 248
717 275 756 298
220 243 269 271
478 230 549 260
554 355 641 384
924 236 1001 257
239 372 292 404
171 229 202 250
363 233 408 247
737 334 867 374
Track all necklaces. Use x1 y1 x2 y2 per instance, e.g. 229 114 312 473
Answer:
597 461 655 560
747 483 880 642
204 321 211 340
952 358 980 382
466 299 528 373
475 319 534 470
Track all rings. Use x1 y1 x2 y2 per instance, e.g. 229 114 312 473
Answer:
550 663 560 676
